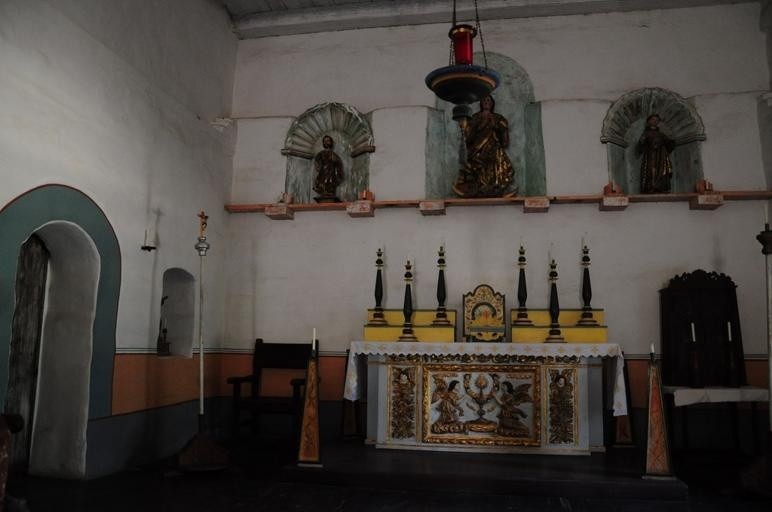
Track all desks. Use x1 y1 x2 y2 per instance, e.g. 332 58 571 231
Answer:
663 384 770 484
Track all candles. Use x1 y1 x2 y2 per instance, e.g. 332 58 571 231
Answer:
145 228 150 245
728 321 732 340
762 203 769 226
311 326 317 350
691 322 696 341
649 342 656 353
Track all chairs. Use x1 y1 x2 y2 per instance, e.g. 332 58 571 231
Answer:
229 339 320 436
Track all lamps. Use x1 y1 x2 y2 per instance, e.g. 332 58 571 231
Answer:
427 0 499 138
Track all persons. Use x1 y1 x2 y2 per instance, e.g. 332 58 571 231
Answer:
311 134 346 202
452 90 518 199
634 115 673 193
548 369 573 440
492 380 530 436
391 366 416 439
429 380 468 435
